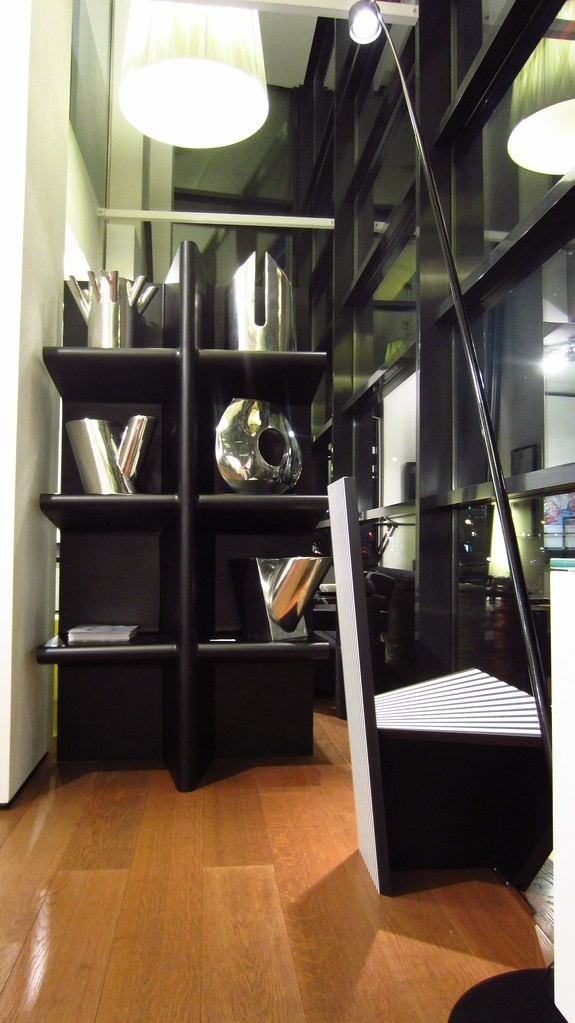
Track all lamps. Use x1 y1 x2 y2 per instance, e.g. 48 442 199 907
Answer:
508 4 575 177
117 1 269 149
349 0 551 767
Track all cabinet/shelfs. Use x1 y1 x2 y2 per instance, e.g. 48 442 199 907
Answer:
40 239 330 790
